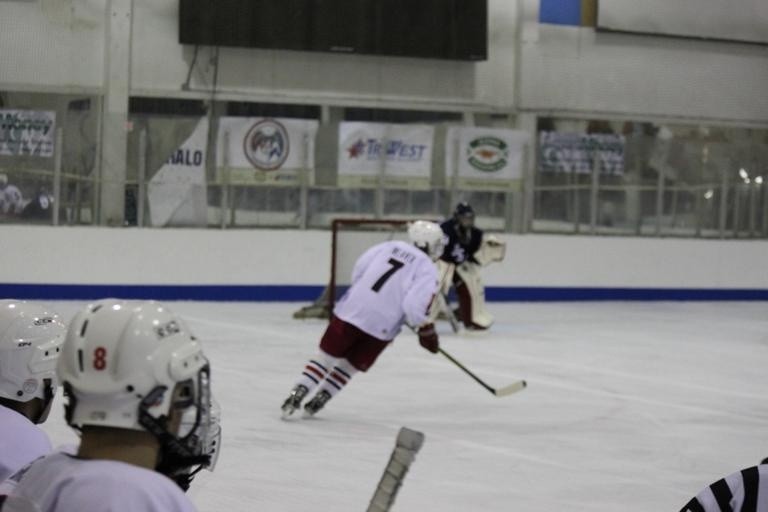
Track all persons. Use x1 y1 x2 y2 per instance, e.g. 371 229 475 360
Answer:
0 174 24 214
0 295 224 511
435 199 494 335
0 295 74 512
675 454 767 512
280 217 449 418
19 179 70 218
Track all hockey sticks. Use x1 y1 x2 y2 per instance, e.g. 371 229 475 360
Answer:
436 283 488 338
404 320 527 397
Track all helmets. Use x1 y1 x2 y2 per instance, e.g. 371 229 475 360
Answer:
1 298 67 424
409 217 449 260
55 298 219 472
452 199 476 234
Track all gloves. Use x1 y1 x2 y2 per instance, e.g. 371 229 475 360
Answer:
417 322 437 353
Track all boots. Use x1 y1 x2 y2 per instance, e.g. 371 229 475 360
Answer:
282 385 332 413
